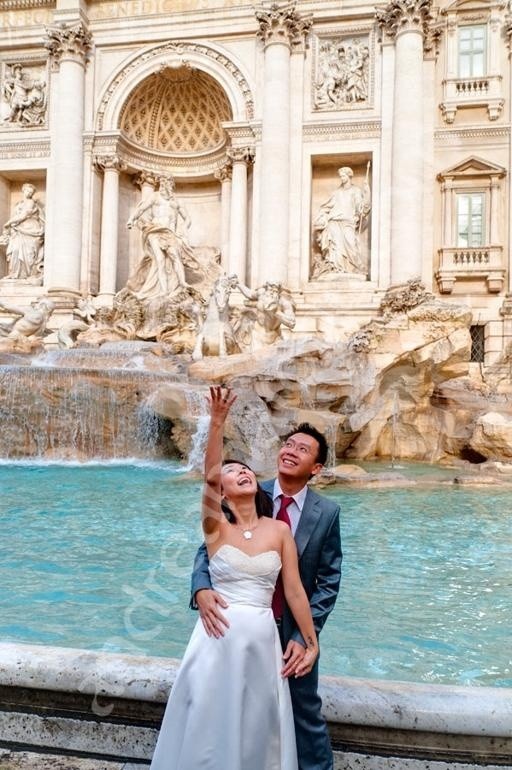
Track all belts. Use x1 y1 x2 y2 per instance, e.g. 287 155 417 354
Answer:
275 617 282 626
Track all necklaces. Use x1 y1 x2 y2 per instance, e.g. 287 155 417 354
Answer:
234 521 259 540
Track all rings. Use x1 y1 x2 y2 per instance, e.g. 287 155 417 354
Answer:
223 399 228 404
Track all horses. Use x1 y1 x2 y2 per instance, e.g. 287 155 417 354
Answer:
189 270 239 362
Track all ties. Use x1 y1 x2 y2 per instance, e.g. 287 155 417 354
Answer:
272 496 295 619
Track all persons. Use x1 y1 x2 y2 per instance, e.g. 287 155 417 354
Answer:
0 294 56 356
2 181 46 281
313 45 369 109
144 383 321 770
233 279 297 350
1 61 49 128
126 169 195 294
187 418 345 770
306 164 371 276
